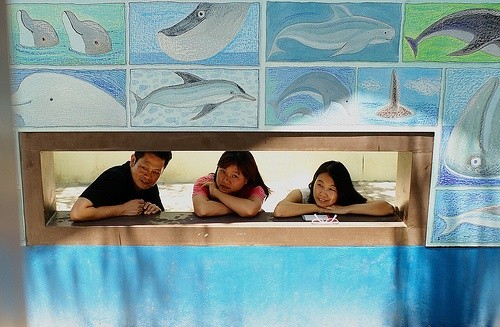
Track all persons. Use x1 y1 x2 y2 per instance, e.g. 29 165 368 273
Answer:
273 160 395 217
190 150 272 218
69 151 173 222
